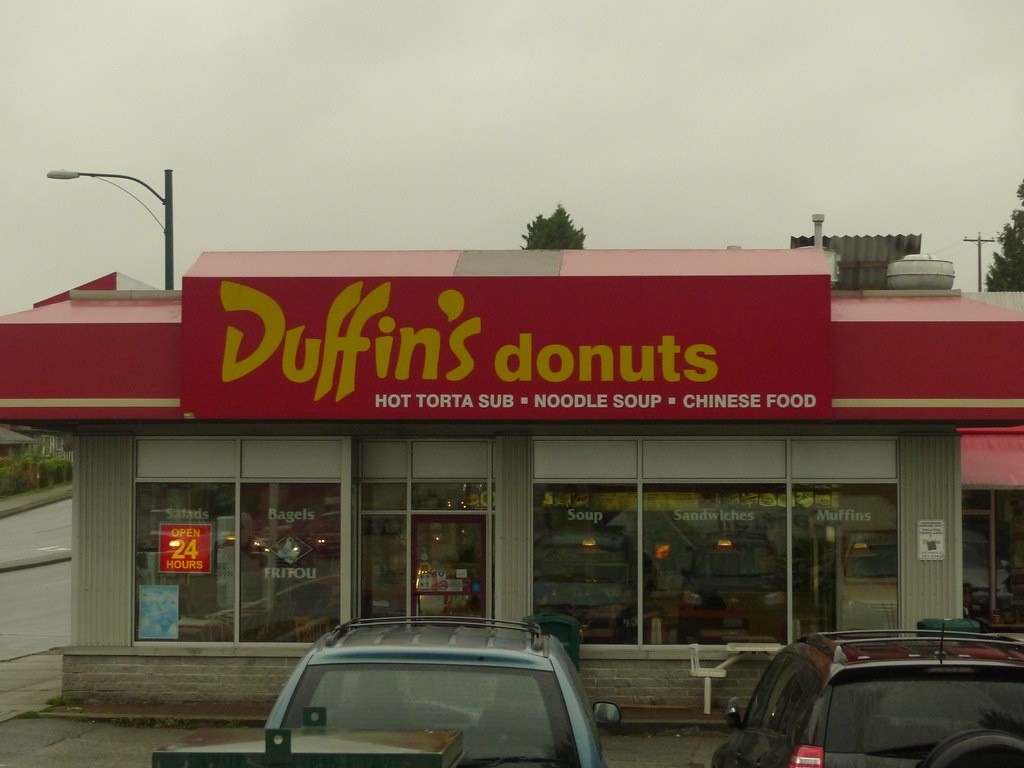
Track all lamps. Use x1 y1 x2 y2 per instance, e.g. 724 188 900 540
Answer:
584 520 596 543
717 520 731 545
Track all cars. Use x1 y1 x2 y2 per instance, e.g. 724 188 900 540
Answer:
534 504 899 642
218 511 342 560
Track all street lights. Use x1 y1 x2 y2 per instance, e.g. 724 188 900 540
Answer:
47 169 174 290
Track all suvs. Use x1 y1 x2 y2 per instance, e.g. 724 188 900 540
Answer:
264 616 622 768
709 629 1024 768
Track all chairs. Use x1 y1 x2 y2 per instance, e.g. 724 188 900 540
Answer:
690 643 727 714
199 601 296 642
294 617 329 643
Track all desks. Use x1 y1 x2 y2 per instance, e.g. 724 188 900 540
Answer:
715 643 781 669
976 617 1024 628
